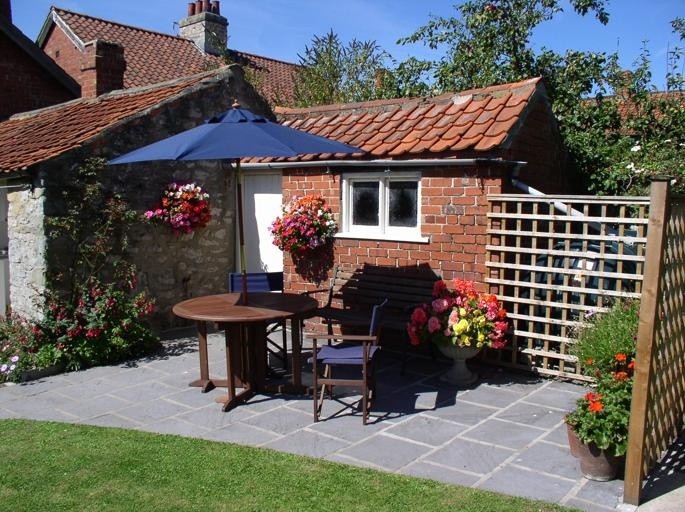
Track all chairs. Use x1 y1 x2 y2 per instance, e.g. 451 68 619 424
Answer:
311 298 389 426
228 271 288 371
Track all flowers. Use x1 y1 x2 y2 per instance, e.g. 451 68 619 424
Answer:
405 278 507 352
270 193 337 259
0 267 155 374
146 181 211 235
569 347 637 452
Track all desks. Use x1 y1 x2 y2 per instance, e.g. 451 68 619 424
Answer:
172 293 318 412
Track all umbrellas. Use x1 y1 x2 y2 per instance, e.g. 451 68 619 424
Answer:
104 98 368 377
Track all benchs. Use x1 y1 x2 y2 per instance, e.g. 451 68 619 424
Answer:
303 268 438 340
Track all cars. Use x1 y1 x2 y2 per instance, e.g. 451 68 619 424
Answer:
517 242 643 313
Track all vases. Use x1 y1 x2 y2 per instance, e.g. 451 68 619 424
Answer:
564 422 622 482
439 346 484 384
